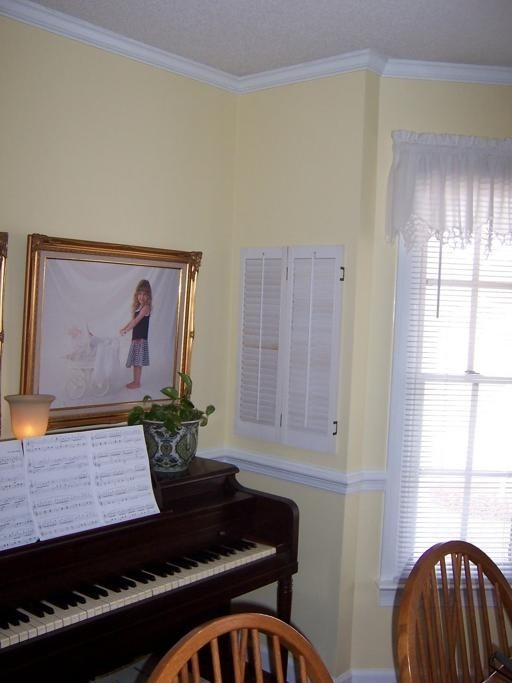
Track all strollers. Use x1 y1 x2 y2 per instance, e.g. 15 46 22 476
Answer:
58 325 124 399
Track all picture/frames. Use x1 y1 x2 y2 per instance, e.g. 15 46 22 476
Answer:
18 231 203 435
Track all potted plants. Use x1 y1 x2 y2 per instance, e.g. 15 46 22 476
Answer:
126 371 216 472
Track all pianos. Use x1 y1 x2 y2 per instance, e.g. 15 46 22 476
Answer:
1 456 298 683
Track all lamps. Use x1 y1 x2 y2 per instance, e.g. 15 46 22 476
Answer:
3 393 57 440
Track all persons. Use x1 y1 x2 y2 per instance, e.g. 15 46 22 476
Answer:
118 277 154 389
